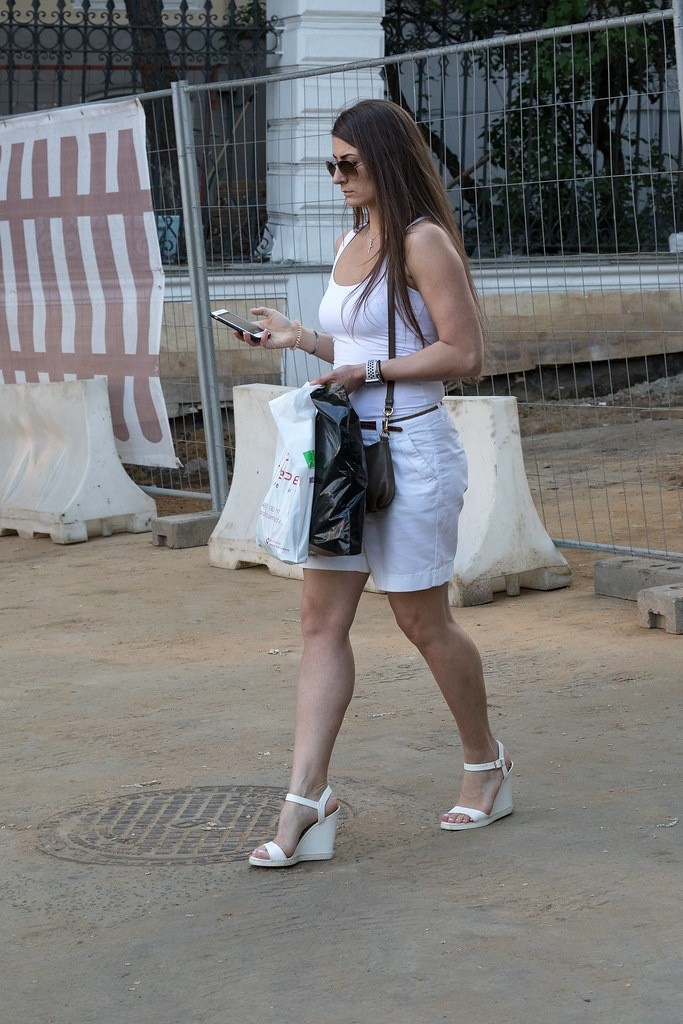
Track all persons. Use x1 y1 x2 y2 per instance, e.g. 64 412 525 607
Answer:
234 100 512 869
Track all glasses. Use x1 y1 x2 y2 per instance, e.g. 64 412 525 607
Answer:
325 160 365 180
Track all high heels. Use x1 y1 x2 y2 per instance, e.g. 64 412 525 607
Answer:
249 785 341 867
439 738 516 830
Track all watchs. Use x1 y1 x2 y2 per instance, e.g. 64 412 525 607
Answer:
364 357 383 386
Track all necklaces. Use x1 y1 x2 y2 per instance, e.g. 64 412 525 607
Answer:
366 225 382 253
309 329 320 355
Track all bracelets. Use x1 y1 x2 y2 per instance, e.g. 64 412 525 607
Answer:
289 319 302 351
378 359 386 383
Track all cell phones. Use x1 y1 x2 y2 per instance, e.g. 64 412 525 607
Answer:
210 308 271 342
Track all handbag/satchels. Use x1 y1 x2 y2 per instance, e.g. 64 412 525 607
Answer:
365 431 395 513
256 383 323 565
308 383 367 557
